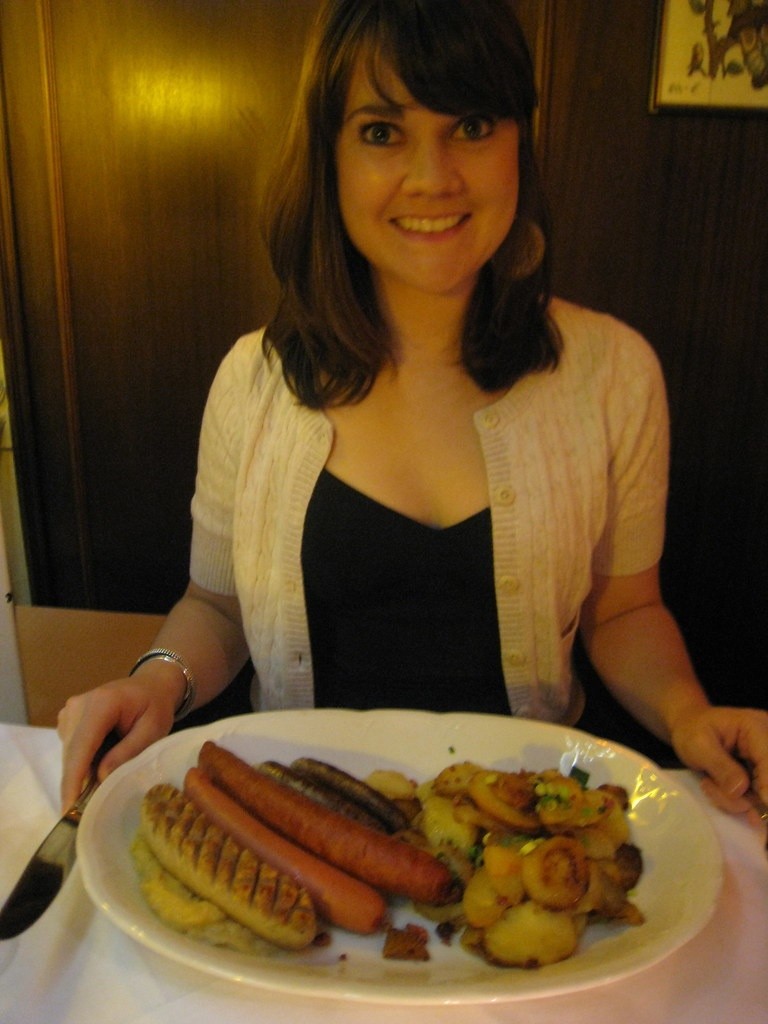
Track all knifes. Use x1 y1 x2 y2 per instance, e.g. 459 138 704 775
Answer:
0 767 120 942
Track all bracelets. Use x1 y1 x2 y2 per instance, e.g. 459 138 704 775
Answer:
129 648 196 722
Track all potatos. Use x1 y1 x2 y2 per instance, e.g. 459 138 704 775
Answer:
362 763 644 965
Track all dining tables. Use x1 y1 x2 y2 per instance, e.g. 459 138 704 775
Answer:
0 721 768 1024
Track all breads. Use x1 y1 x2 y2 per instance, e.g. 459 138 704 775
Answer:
143 783 319 951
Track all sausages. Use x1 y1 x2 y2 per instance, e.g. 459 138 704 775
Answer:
184 740 462 934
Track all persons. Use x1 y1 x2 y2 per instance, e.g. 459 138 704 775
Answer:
58 1 768 837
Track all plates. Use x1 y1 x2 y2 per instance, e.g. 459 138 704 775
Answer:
76 706 723 1007
